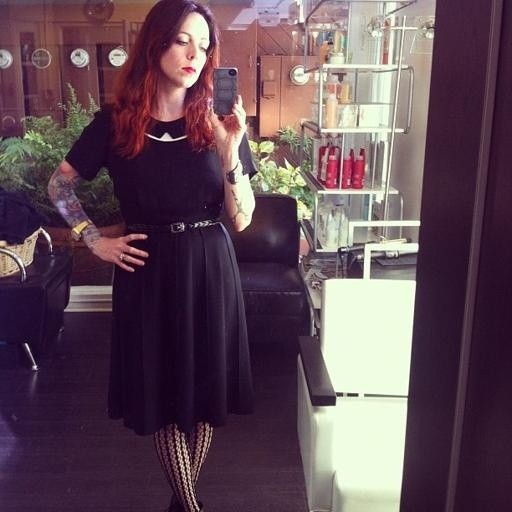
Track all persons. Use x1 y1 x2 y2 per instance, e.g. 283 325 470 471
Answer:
45 0 260 512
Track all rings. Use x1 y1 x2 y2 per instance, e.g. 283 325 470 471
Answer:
119 252 124 261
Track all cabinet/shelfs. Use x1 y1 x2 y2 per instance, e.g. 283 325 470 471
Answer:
300 14 420 253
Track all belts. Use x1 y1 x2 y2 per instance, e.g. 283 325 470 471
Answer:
124 217 222 235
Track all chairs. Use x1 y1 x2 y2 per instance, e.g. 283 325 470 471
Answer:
1 188 74 370
222 194 307 347
296 279 416 511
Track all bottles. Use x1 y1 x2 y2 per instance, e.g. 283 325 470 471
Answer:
323 76 354 129
326 156 365 189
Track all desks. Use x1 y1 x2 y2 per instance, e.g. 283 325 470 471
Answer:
300 252 417 337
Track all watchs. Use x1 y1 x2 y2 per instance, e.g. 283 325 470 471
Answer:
70 217 93 241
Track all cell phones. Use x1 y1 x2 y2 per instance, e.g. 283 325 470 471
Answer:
212 67 239 115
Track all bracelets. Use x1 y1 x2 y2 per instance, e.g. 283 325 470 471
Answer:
223 160 243 184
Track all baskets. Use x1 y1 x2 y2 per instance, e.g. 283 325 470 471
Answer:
0 227 42 278
